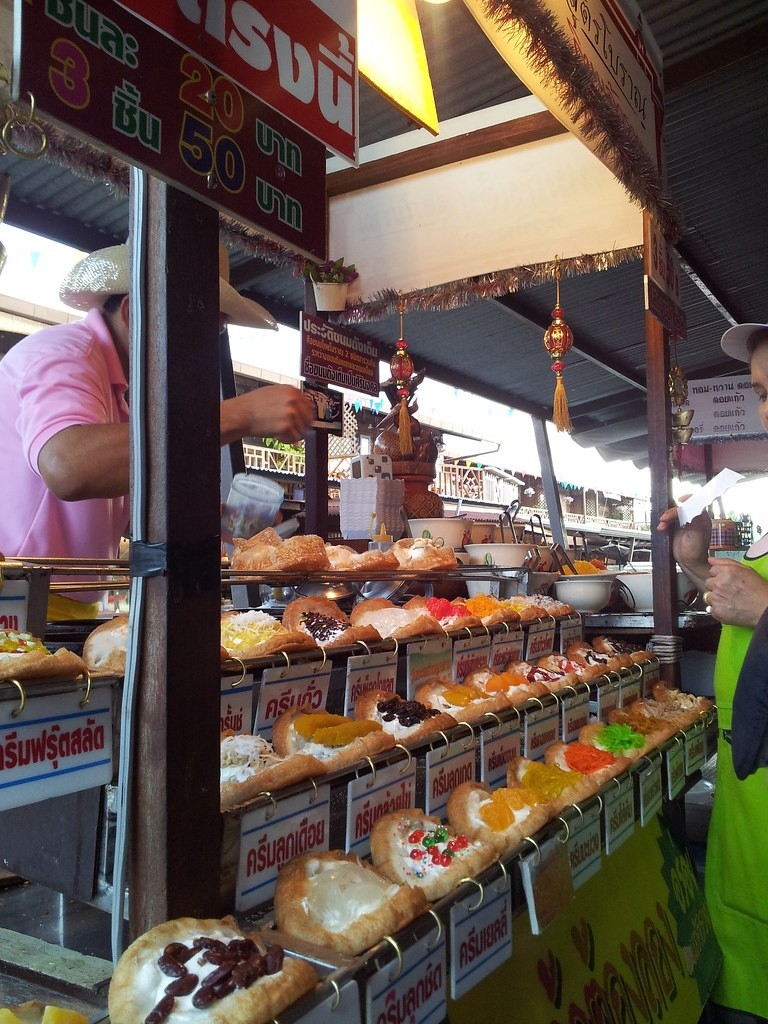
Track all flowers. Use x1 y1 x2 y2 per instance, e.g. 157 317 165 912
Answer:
291 256 359 285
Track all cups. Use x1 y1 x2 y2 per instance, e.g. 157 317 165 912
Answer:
222 472 285 545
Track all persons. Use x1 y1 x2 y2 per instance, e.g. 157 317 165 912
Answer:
0 230 315 623
308 393 339 423
656 323 768 1024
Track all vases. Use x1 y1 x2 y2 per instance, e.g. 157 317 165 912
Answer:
312 282 350 312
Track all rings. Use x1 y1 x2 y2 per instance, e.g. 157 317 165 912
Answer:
703 590 713 607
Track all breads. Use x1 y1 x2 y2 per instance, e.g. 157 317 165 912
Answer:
107 916 316 1024
0 627 88 681
222 527 712 957
1 1001 87 1024
82 615 129 676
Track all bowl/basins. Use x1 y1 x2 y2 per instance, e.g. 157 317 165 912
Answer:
406 518 699 614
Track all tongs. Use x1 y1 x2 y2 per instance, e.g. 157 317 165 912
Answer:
497 501 589 576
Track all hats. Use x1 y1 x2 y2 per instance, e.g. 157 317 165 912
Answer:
720 323 768 363
59 233 279 331
